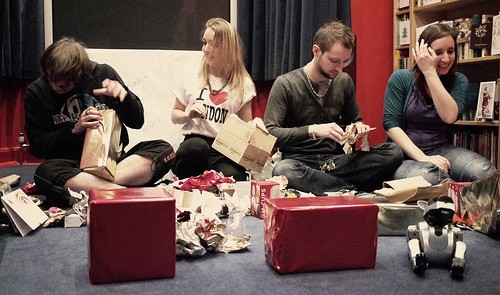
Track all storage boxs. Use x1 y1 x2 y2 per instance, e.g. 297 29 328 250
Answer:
376 203 425 236
89 187 176 285
251 180 279 219
211 114 277 173
263 198 380 275
80 109 121 181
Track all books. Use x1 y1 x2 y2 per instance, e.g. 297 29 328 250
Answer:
396 0 500 168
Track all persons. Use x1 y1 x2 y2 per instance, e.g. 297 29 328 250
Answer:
23 36 176 205
170 19 269 182
382 24 498 190
264 20 403 198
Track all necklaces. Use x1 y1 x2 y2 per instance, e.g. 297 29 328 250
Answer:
205 67 234 96
305 63 333 98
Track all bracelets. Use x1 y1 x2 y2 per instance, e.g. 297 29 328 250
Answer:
312 124 317 139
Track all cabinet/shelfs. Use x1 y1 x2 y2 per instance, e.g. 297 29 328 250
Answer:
393 0 500 174
